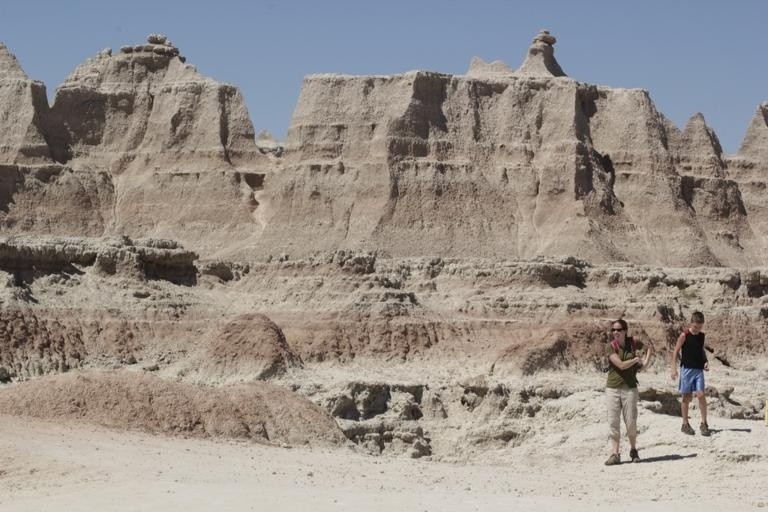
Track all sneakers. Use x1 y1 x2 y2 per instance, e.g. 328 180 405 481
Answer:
630 449 640 462
700 422 710 437
681 422 695 435
604 452 621 466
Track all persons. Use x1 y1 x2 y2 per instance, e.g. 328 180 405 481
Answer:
604 320 642 465
670 312 710 436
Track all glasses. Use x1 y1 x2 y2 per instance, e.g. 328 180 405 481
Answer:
611 327 624 332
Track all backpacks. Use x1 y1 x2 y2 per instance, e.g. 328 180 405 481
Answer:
611 336 643 372
